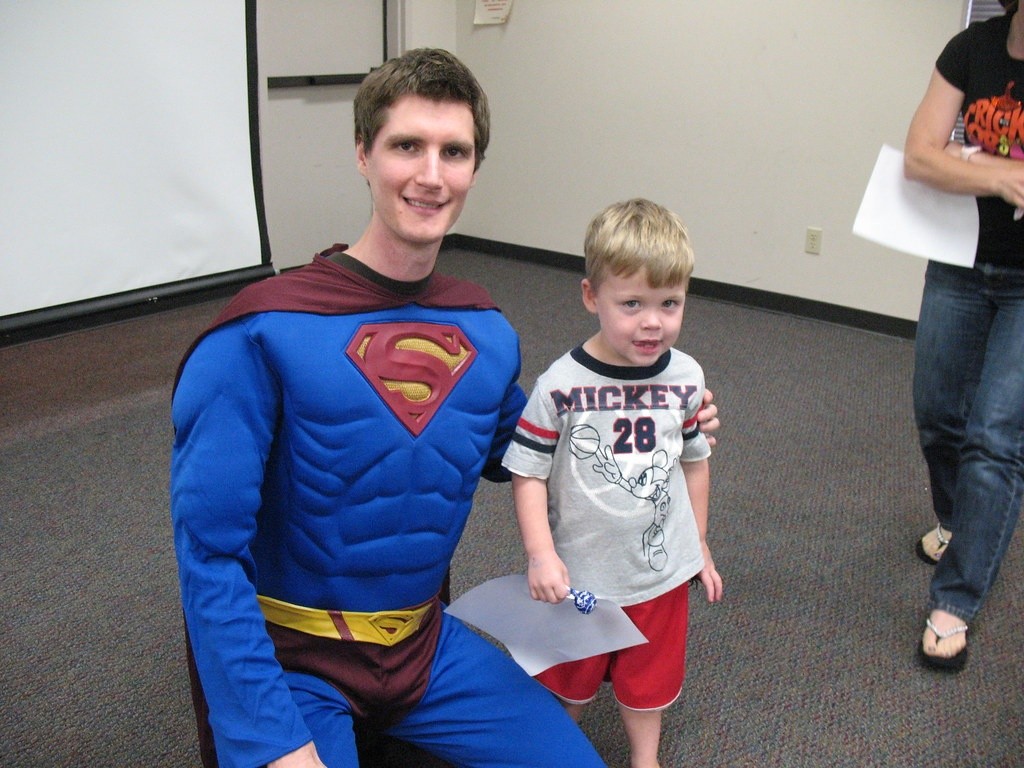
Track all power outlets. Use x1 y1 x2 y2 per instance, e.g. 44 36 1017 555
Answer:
804 227 823 254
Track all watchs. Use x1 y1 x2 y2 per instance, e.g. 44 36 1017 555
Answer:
961 142 983 163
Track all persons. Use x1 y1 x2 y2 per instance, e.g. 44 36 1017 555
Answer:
499 196 725 768
166 47 607 767
901 0 1024 674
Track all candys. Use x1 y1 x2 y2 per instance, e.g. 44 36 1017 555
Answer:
566 587 596 616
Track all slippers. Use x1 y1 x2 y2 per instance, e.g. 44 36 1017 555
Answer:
919 611 969 671
917 522 953 564
